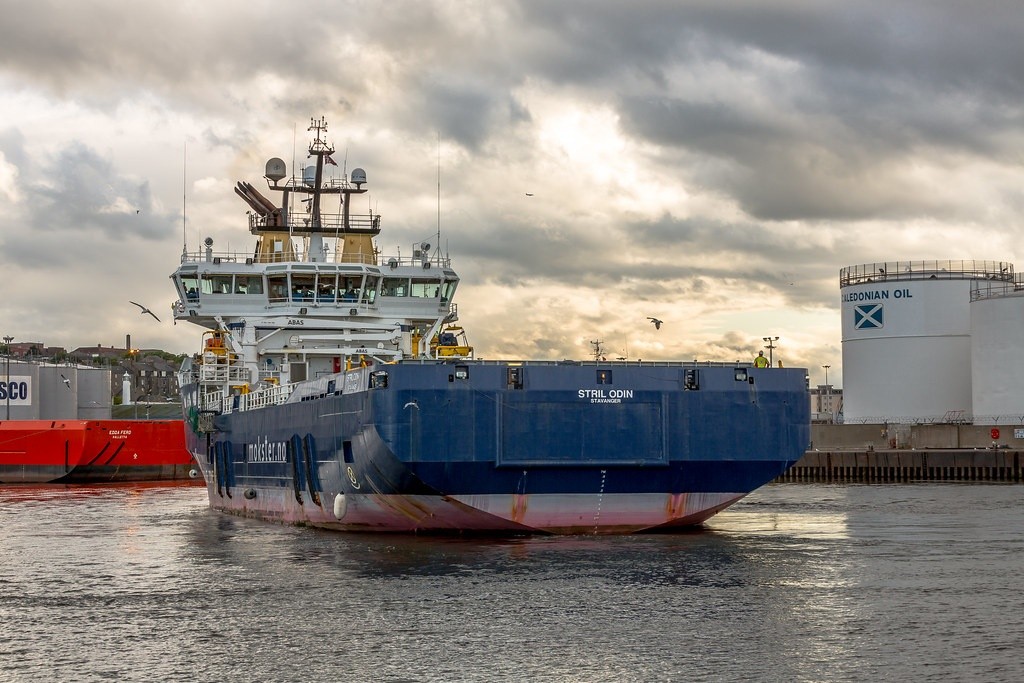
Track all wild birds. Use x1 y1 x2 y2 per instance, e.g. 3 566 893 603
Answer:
163 395 175 402
403 401 420 410
647 317 664 330
815 448 819 451
129 300 162 322
136 209 139 214
526 193 534 197
60 374 71 389
93 401 102 406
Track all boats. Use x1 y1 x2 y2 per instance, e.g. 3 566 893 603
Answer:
169 116 811 531
0 334 204 482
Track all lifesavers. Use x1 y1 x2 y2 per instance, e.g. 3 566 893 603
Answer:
990 428 999 439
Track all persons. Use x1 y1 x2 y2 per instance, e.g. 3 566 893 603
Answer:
435 287 439 297
183 282 188 295
301 286 313 297
754 350 770 369
778 360 784 369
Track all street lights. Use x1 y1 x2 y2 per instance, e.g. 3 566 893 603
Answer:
822 365 830 391
130 349 139 420
763 337 779 368
